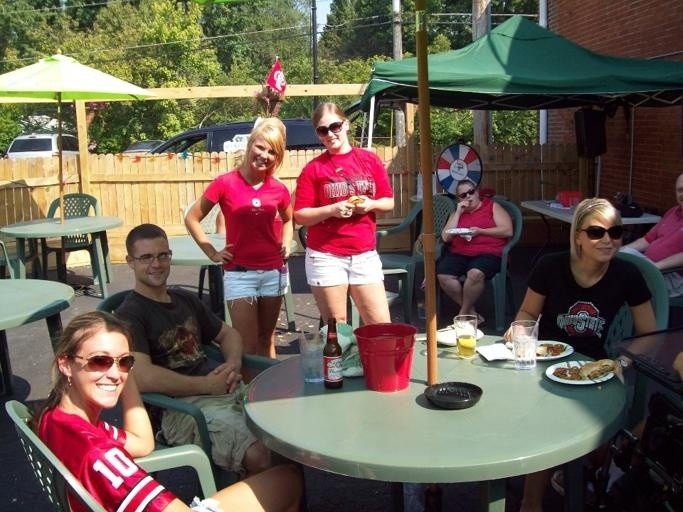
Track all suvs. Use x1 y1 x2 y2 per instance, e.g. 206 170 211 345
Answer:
2 133 83 156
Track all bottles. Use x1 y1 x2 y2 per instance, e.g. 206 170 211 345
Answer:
322 317 343 389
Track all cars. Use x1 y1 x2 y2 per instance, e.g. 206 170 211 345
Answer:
122 139 163 153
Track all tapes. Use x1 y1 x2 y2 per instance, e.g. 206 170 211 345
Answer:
347 196 363 206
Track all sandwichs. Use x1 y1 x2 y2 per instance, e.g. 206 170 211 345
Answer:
580 359 615 380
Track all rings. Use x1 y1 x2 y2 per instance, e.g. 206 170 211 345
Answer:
343 211 350 215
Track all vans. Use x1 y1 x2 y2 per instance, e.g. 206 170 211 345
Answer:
143 98 361 152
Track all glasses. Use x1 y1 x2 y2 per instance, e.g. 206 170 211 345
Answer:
70 353 135 372
581 225 624 239
458 188 476 199
130 250 172 264
315 119 344 137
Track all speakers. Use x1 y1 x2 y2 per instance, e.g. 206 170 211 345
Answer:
574 109 607 159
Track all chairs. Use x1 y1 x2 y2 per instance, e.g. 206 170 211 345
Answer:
5 400 218 512
436 199 525 331
183 200 221 301
299 226 410 340
42 193 101 287
94 289 279 488
374 196 456 325
604 252 670 413
0 240 31 279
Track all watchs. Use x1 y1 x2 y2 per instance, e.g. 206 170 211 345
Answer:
613 355 628 368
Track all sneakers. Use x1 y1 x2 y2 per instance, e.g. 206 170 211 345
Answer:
551 468 622 509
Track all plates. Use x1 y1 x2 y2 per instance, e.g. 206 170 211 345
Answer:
535 339 574 361
424 382 482 409
544 361 614 385
445 227 471 235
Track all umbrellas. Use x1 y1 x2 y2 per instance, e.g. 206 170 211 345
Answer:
0 55 155 263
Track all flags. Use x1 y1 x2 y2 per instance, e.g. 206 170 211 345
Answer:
264 58 288 94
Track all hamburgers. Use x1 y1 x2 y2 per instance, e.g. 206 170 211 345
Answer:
348 195 363 205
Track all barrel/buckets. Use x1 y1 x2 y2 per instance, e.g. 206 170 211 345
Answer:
355 323 417 391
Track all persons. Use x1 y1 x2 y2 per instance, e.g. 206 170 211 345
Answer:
505 198 657 498
109 224 275 478
185 114 290 385
622 172 683 299
292 102 395 329
437 178 513 325
39 311 305 512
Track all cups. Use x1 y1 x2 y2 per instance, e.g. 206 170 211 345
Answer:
299 333 324 385
453 314 477 360
510 320 538 371
